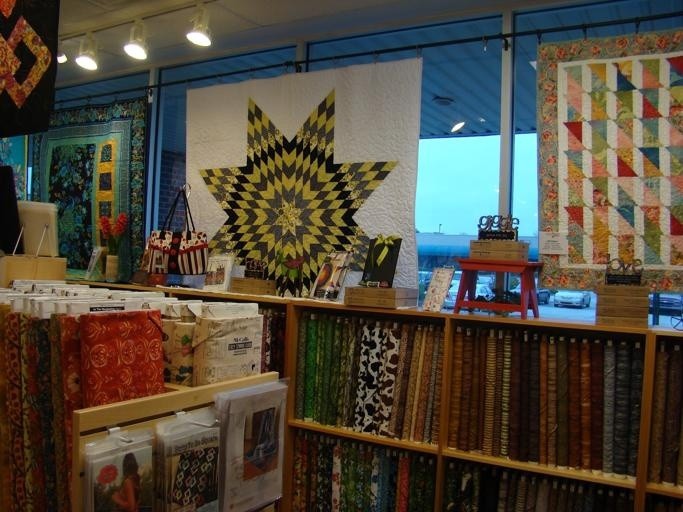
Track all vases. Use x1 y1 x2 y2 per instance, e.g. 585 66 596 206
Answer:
105 253 121 283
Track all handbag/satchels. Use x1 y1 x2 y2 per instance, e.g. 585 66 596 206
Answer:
139 230 207 275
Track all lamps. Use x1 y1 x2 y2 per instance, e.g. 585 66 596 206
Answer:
73 0 217 72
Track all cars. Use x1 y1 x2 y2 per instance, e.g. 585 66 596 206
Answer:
418 270 592 312
648 293 683 316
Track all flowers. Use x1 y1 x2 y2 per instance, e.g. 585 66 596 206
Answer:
99 213 129 256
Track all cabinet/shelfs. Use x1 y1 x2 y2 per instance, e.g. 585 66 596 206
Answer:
0 277 683 510
